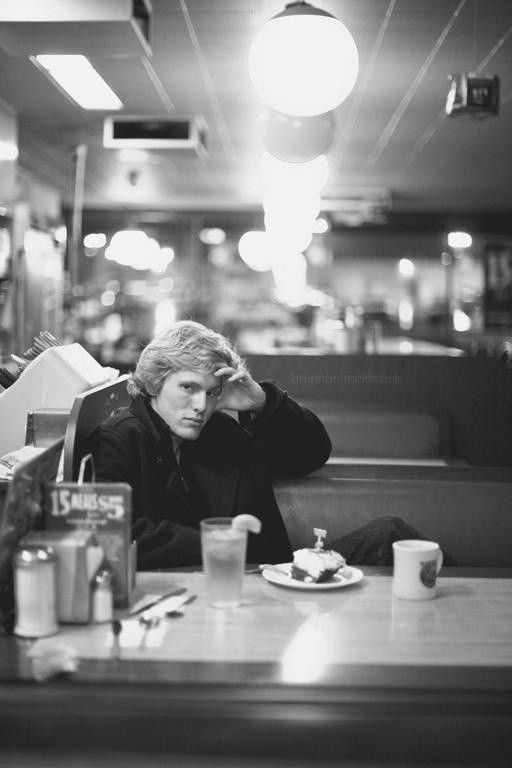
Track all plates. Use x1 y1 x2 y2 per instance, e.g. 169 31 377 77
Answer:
262 562 363 592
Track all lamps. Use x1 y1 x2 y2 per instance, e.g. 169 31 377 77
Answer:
240 1 360 306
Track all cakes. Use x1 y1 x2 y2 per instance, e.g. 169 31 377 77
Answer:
291 546 346 581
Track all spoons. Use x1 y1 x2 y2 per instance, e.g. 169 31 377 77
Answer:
165 595 196 617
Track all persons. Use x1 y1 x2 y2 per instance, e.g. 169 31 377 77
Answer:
87 322 431 577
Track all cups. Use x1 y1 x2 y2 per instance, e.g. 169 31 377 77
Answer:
200 516 250 609
392 539 444 601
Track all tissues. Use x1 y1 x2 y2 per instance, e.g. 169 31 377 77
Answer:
19 529 103 625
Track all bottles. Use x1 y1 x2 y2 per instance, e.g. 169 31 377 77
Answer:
10 544 60 637
89 570 113 622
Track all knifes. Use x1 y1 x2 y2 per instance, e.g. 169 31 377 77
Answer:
129 586 186 614
